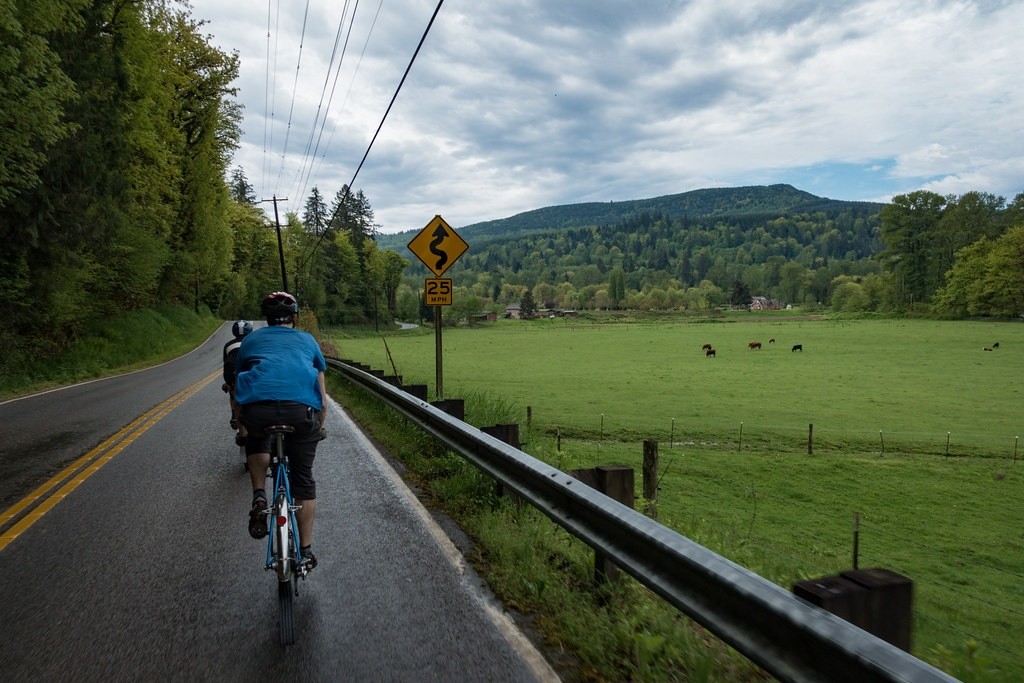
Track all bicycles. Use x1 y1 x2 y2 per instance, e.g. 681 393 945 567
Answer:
244 406 327 647
222 373 253 472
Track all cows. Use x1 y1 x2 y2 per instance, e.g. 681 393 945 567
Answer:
983 342 999 351
748 342 762 349
701 343 716 357
767 337 776 343
792 344 802 352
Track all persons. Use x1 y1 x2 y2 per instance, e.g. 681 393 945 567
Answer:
223 321 253 430
235 292 327 568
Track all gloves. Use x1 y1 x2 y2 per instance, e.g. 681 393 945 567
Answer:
236 433 247 447
222 384 230 393
320 429 327 440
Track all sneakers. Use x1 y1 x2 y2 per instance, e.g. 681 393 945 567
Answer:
301 547 318 569
248 496 268 539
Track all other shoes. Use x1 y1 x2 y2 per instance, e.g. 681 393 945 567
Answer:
230 419 239 430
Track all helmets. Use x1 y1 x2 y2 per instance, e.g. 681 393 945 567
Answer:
260 291 299 324
232 320 253 338
